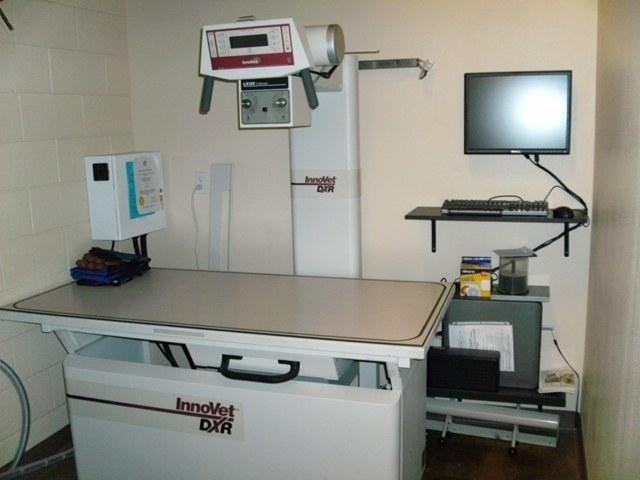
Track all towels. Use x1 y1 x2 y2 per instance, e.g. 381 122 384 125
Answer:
70 246 151 287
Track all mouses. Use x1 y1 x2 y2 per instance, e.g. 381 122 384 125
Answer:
554 206 575 221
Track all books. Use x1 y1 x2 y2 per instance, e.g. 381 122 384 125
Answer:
539 368 576 394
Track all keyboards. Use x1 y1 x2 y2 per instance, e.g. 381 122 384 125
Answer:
440 197 548 217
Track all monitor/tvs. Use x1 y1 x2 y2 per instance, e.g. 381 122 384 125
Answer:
463 69 572 156
